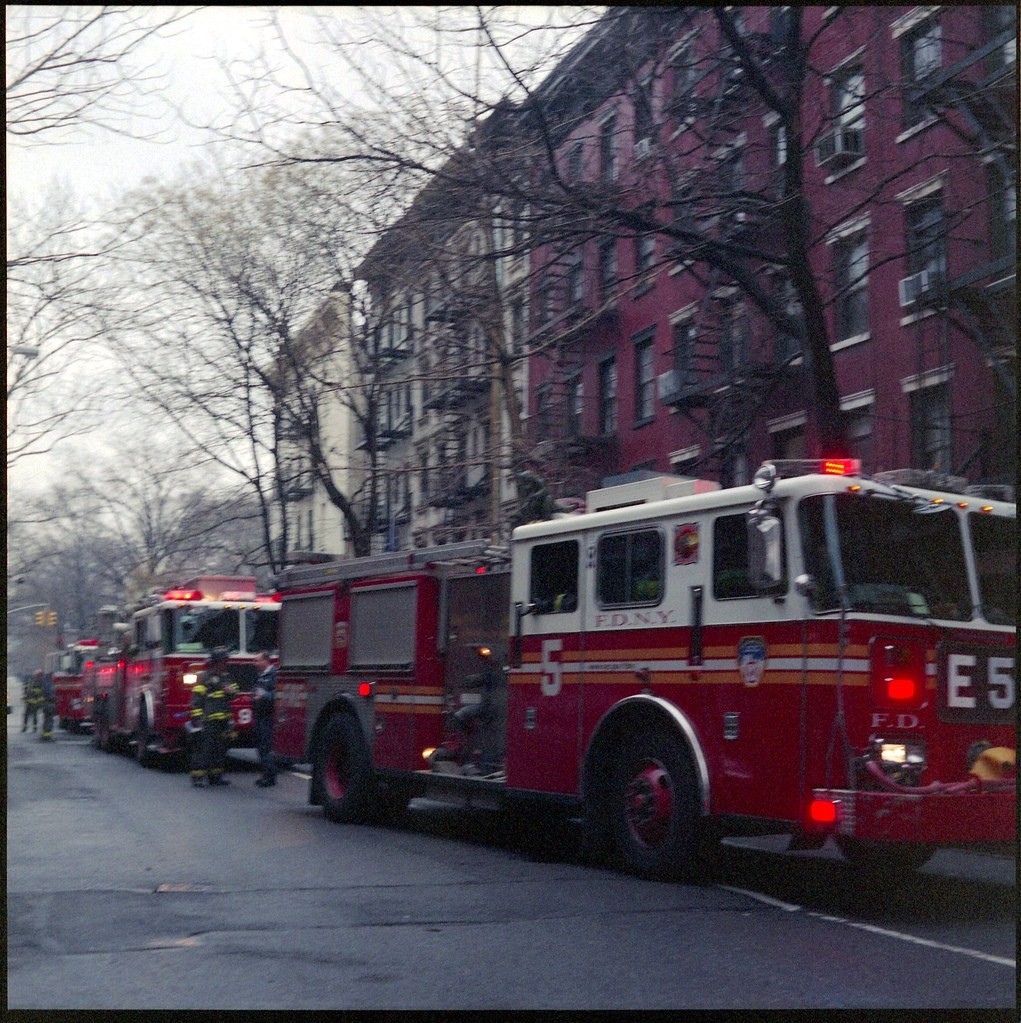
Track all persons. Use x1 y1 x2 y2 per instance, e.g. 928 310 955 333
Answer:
21 671 56 742
250 652 277 788
189 646 240 788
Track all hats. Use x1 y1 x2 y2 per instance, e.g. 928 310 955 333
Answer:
212 646 229 661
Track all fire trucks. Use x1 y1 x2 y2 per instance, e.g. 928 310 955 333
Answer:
44 576 283 769
266 460 1021 884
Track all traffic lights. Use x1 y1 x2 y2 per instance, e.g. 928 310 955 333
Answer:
33 611 44 629
47 611 60 629
